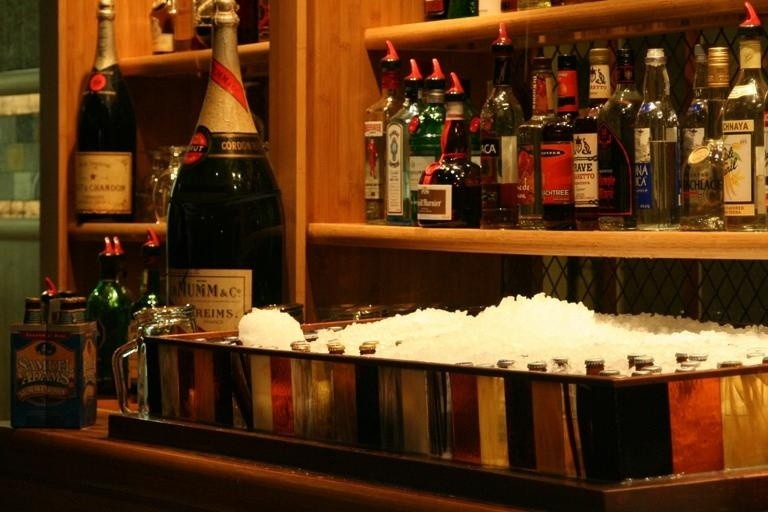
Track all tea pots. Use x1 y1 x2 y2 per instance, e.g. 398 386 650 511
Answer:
112 302 197 422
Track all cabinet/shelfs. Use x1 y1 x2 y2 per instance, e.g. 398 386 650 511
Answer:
0 1 768 511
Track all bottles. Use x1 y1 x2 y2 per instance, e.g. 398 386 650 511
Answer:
64 1 138 226
164 0 289 336
359 0 767 227
10 288 97 432
290 340 379 359
457 347 767 377
90 229 157 399
148 2 270 55
